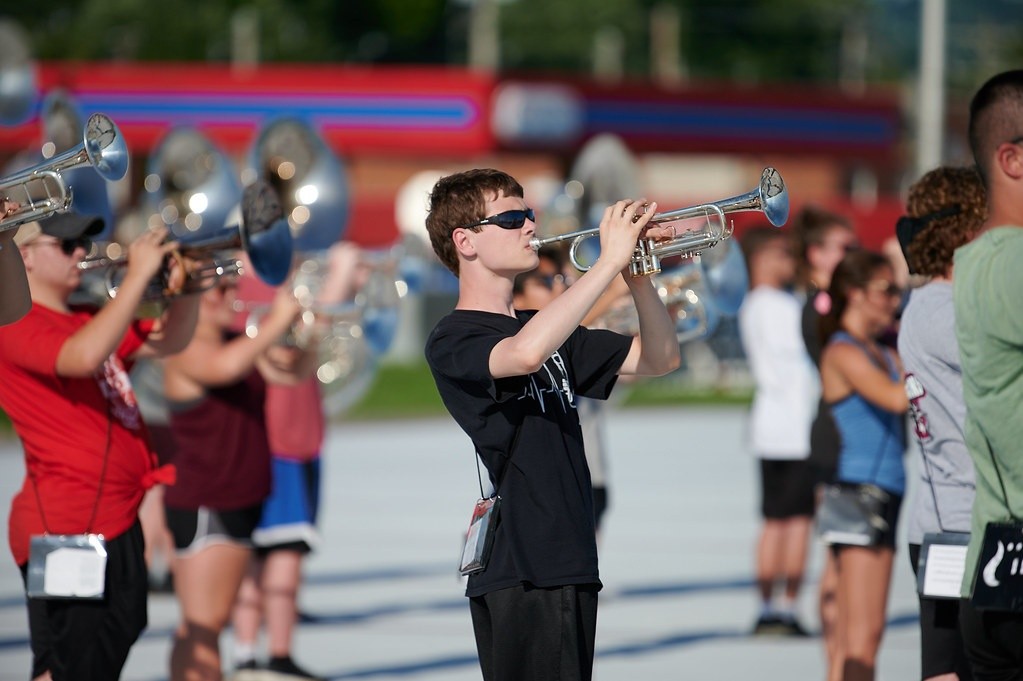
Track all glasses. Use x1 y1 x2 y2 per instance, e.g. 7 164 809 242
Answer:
447 208 536 238
26 236 92 256
854 280 900 296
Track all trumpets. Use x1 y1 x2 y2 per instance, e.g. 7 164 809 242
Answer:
1 26 435 387
531 170 789 273
588 226 748 343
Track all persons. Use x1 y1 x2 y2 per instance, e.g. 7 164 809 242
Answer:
823 250 911 681
159 250 300 681
0 212 184 680
953 69 1023 681
233 242 361 681
423 168 681 681
800 205 862 660
897 164 987 681
736 227 822 634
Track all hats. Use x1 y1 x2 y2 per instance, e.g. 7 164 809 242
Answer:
12 212 105 246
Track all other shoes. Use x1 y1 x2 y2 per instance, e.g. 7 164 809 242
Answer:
235 654 321 681
755 614 814 635
